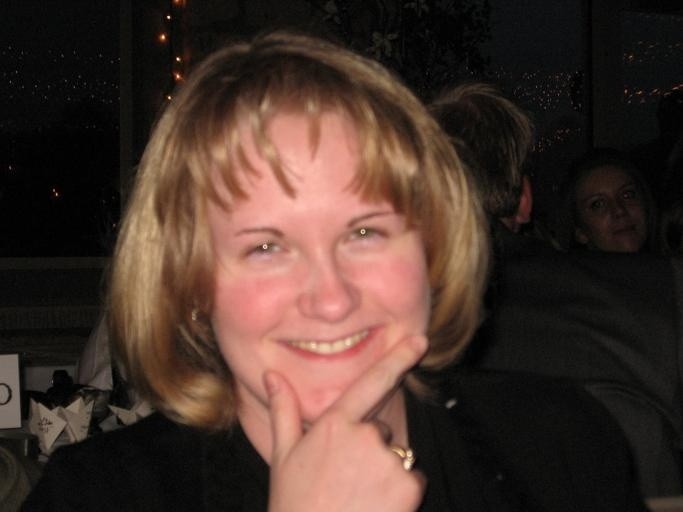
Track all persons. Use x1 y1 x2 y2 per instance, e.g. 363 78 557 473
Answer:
17 32 647 512
565 165 652 255
420 84 683 512
